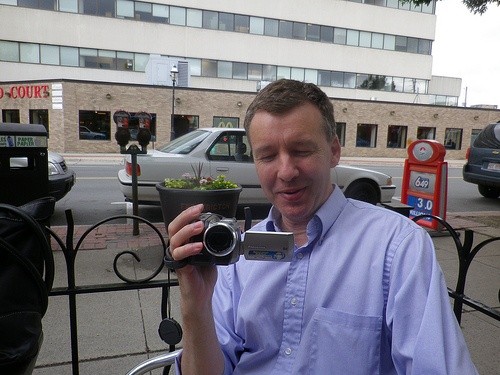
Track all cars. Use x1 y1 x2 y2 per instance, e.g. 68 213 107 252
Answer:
80 126 108 140
357 138 369 147
129 129 156 141
118 128 396 219
10 150 76 202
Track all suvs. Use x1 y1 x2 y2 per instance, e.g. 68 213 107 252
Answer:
463 121 500 198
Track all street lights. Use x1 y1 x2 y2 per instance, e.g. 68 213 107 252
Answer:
169 65 179 142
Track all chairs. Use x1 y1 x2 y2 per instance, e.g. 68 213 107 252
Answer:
235 143 249 161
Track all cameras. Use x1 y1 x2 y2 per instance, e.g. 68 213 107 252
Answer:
188 212 295 267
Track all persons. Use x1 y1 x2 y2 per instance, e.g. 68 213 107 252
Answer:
168 79 476 373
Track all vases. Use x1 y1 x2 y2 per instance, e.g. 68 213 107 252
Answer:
156 183 242 233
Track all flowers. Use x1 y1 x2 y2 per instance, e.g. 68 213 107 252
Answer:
164 162 238 190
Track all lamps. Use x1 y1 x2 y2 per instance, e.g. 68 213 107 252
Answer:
343 107 348 113
43 91 49 97
474 115 478 120
434 113 438 118
5 91 11 97
390 111 395 116
106 93 111 99
237 102 243 107
176 97 181 103
